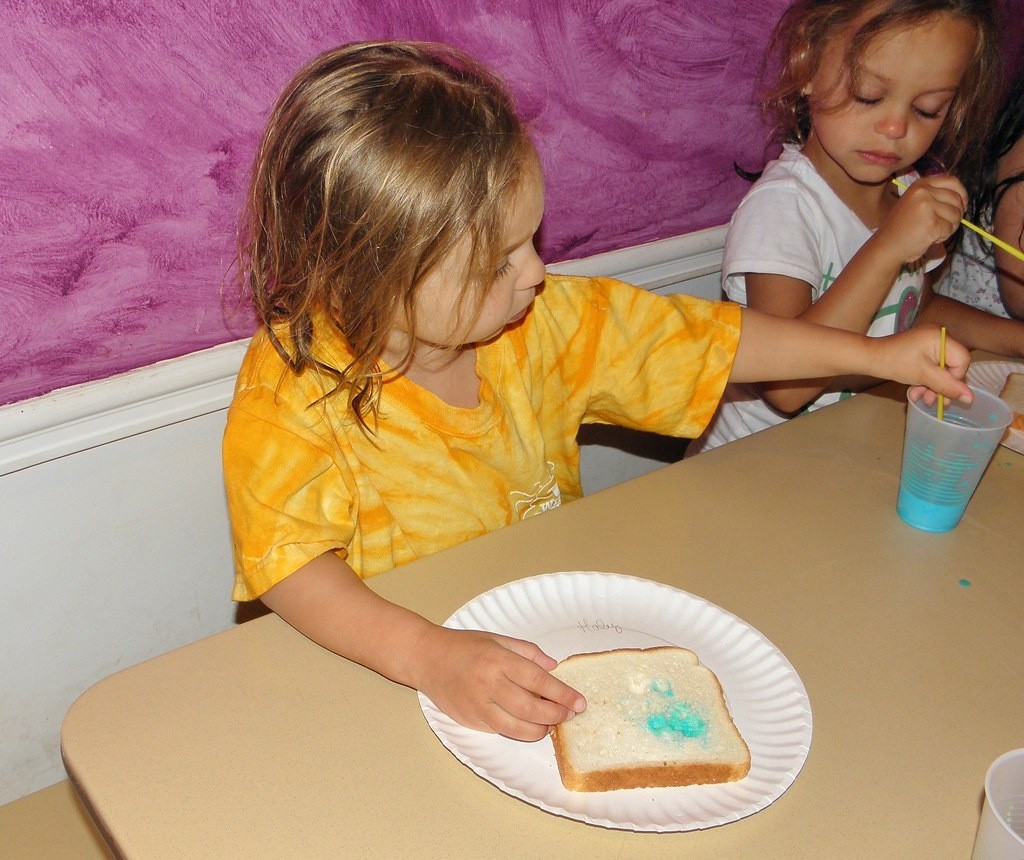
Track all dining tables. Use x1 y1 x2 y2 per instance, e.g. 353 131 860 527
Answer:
60 347 1024 860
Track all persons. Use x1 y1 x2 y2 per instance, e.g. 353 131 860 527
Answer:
683 0 1024 458
221 39 974 741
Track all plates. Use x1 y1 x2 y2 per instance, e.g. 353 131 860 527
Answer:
415 570 814 835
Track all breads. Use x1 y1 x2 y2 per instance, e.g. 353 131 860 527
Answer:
999 371 1024 433
540 646 751 791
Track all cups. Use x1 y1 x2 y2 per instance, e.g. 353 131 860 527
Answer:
971 748 1024 860
895 380 1015 533
